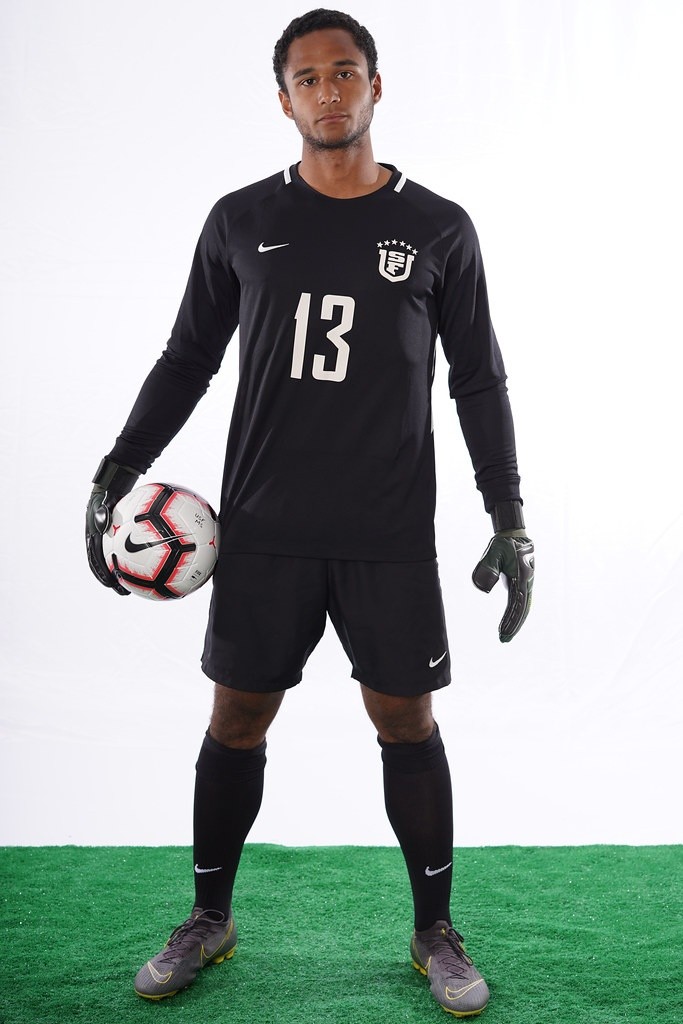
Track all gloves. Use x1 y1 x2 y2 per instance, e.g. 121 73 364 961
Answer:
87 484 132 596
472 526 534 643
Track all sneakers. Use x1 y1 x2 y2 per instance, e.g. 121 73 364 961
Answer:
134 905 237 1000
409 919 490 1017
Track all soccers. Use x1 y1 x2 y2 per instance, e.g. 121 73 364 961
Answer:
102 482 220 602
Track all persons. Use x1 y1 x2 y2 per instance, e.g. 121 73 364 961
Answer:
84 11 535 1017
